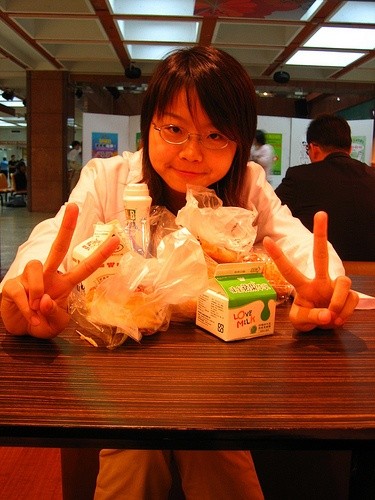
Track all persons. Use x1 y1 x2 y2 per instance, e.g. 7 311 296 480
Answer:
68 141 81 194
0 154 27 192
0 45 360 500
272 115 375 261
251 131 273 180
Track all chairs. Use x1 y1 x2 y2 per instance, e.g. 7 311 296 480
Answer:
0 173 28 206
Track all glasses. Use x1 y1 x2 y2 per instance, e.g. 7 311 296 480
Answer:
151 119 235 150
305 143 318 155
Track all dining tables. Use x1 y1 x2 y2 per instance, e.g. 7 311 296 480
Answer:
0 257 375 500
